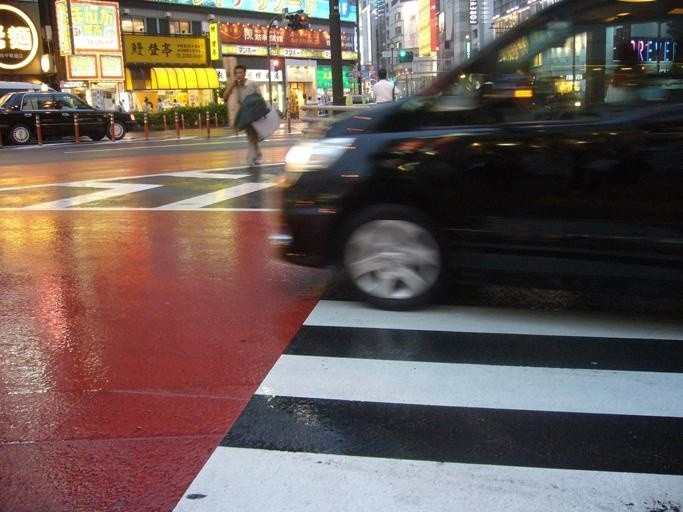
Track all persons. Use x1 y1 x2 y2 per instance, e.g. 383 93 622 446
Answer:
173 99 180 108
143 95 164 114
273 99 278 111
286 97 288 113
112 99 125 113
223 65 262 168
371 68 396 105
306 96 313 106
322 88 331 113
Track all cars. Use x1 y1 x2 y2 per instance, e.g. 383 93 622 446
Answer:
0 92 140 143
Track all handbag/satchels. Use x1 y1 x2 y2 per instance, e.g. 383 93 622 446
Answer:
250 110 281 139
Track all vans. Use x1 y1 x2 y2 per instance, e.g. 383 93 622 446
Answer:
268 0 683 326
0 80 58 98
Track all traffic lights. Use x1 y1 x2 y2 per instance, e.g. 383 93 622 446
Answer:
399 50 406 62
286 13 308 30
